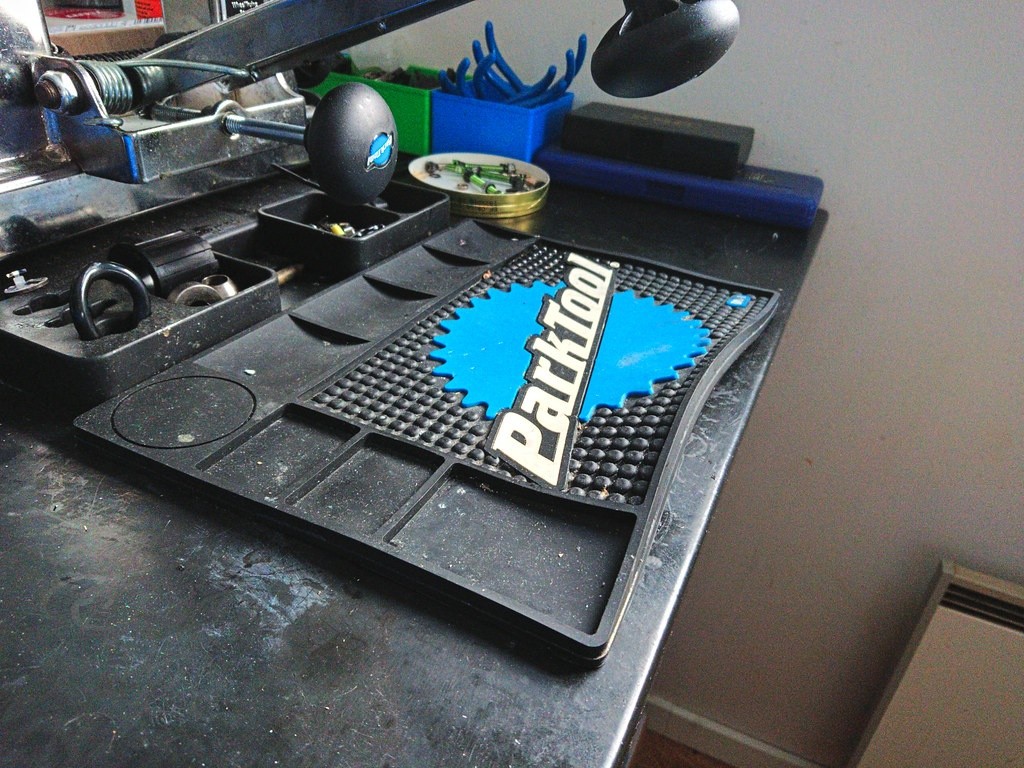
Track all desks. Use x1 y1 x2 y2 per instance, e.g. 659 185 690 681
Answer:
1 209 831 768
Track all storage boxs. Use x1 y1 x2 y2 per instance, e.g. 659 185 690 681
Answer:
310 64 574 164
